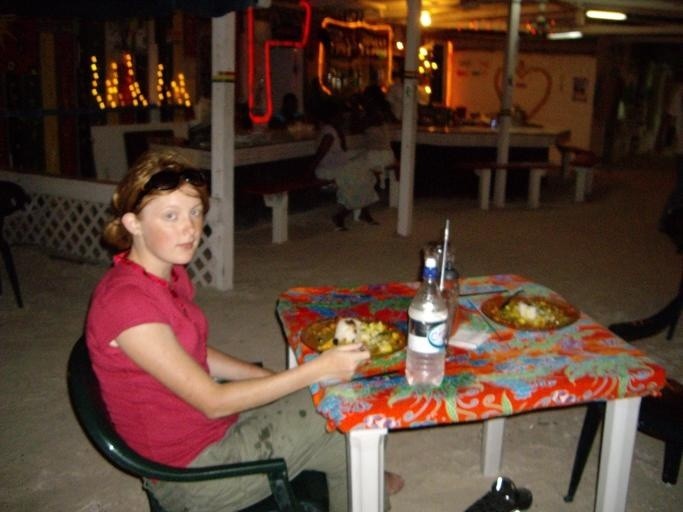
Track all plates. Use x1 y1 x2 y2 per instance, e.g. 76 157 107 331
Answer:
299 317 407 359
480 293 580 333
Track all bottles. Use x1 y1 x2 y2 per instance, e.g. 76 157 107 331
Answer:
422 240 456 265
325 30 387 93
404 256 449 388
439 261 462 335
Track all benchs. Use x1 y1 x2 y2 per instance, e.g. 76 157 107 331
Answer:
561 145 608 205
466 161 562 210
250 156 403 245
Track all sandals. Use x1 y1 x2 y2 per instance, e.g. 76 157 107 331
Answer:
466 477 534 512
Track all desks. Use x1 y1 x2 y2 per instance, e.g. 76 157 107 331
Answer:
389 126 572 192
153 134 339 230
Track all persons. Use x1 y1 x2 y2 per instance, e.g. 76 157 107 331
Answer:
233 65 403 233
85 149 370 512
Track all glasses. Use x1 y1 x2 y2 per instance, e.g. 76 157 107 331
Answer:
132 168 207 210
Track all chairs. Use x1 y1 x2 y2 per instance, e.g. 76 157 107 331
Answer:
61 326 297 510
562 287 683 504
0 182 34 310
